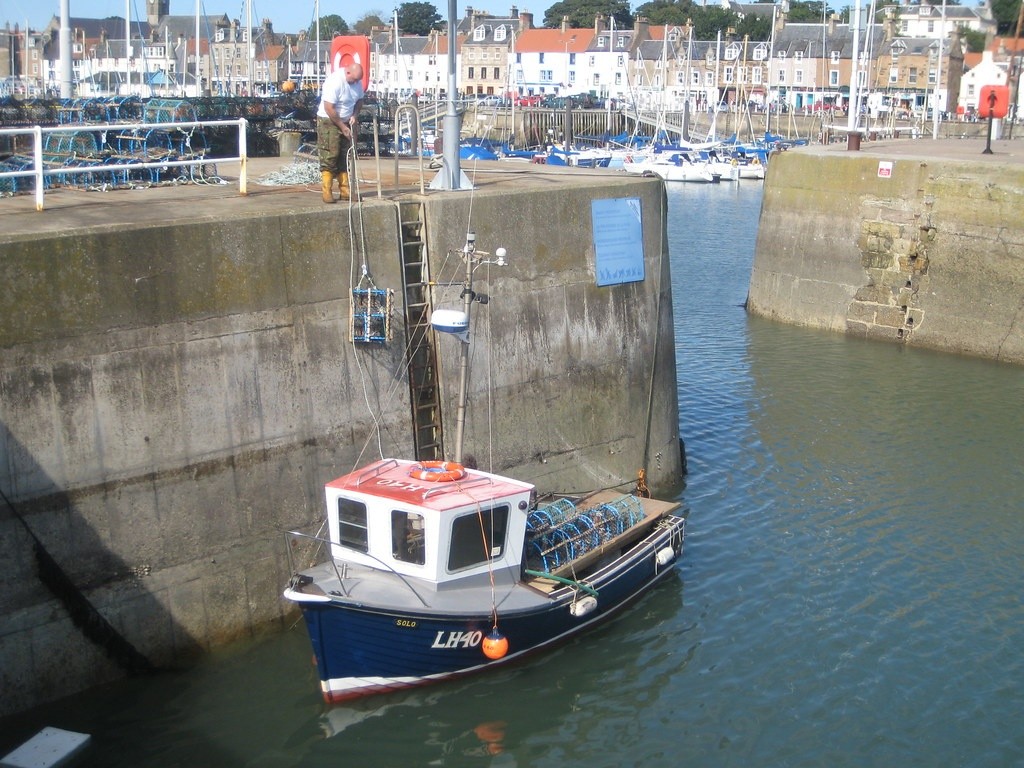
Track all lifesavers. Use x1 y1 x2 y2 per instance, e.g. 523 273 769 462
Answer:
731 159 737 167
333 44 360 73
752 158 760 166
409 460 466 482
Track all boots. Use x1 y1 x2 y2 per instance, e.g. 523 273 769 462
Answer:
322 170 336 202
337 171 362 201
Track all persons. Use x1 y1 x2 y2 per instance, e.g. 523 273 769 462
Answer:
317 63 364 203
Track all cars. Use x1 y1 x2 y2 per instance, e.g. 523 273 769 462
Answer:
909 106 952 119
464 93 486 102
514 95 540 106
710 99 728 113
568 92 592 109
4 74 51 98
436 93 447 103
541 96 572 109
732 99 762 113
474 94 500 106
417 92 432 103
763 98 793 113
596 97 617 110
228 84 259 98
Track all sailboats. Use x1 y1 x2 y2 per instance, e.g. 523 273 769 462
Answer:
0 0 973 182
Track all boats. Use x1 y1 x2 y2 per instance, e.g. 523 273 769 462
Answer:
284 157 685 705
